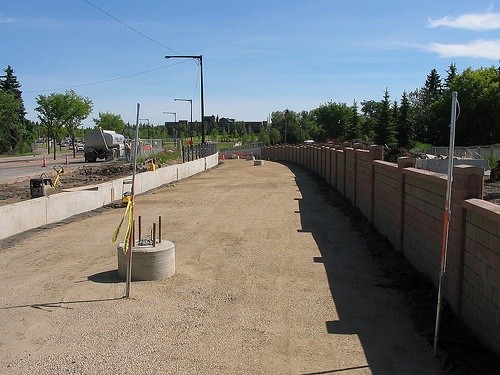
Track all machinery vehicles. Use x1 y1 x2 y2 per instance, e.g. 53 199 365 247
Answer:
84 129 130 161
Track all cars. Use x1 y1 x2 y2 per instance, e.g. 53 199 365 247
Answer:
57 136 85 152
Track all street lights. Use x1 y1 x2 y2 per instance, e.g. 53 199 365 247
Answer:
139 54 205 146
